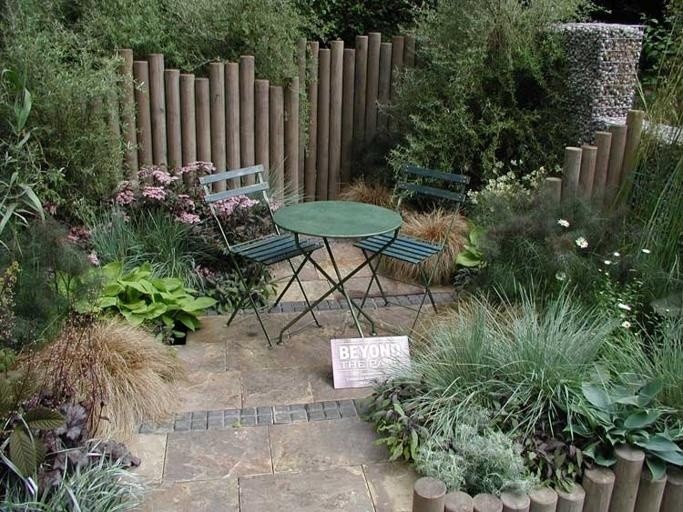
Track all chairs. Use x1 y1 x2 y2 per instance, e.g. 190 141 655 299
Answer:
352 163 471 337
198 162 324 350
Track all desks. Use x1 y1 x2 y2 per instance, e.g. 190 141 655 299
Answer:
269 200 402 346
